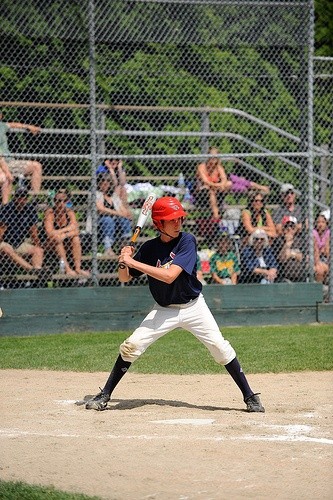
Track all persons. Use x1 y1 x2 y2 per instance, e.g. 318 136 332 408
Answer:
86 197 265 412
41 193 86 276
0 190 52 276
196 148 233 220
91 158 133 258
236 183 333 284
210 235 241 285
0 112 50 206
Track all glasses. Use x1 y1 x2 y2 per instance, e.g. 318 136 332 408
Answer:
53 198 63 202
110 158 120 162
286 191 294 194
283 224 292 228
256 238 264 241
255 199 264 203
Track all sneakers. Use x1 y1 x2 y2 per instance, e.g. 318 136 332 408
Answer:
245 393 265 412
85 387 110 411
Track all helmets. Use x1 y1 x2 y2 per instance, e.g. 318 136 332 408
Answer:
151 197 187 230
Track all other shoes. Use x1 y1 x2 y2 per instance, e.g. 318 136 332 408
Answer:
67 270 90 277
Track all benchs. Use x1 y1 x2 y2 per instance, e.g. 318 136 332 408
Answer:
0 190 280 280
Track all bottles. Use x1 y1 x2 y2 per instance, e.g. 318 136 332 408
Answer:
59 260 66 275
19 172 24 186
184 188 190 208
178 172 184 188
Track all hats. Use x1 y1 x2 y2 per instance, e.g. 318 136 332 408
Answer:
280 183 295 191
282 215 298 229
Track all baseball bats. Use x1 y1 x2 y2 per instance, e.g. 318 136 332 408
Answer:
120 193 155 269
